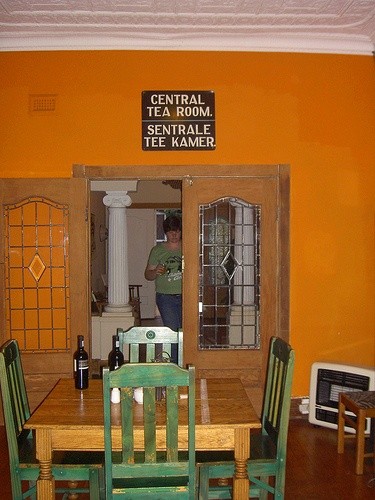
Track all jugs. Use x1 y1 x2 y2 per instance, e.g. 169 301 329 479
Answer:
150 351 174 402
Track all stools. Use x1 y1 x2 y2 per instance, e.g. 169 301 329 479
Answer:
338 391 375 475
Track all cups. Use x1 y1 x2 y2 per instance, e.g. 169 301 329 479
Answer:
159 259 167 276
133 387 143 405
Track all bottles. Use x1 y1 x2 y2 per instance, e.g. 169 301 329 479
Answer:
73 335 89 390
108 335 124 371
111 388 121 404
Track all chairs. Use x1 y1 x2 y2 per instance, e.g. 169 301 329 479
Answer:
101 363 197 500
200 335 294 500
0 339 101 500
115 327 185 368
92 274 142 325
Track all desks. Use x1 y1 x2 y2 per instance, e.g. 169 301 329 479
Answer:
23 377 262 500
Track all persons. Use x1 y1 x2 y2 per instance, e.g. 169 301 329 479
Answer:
145 215 182 364
